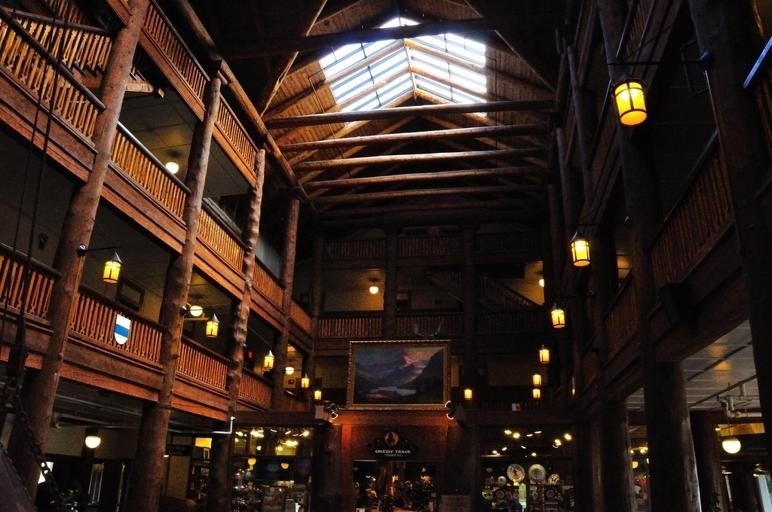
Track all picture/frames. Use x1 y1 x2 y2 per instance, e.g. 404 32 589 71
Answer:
346 339 452 412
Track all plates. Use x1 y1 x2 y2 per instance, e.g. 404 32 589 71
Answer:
527 463 547 482
505 463 525 483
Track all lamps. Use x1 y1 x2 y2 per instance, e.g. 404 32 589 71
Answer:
281 368 322 400
607 48 712 127
244 344 276 369
532 373 542 398
76 243 122 283
539 344 550 363
722 420 741 455
179 306 220 338
550 304 566 329
569 215 632 268
369 278 379 294
85 431 101 449
